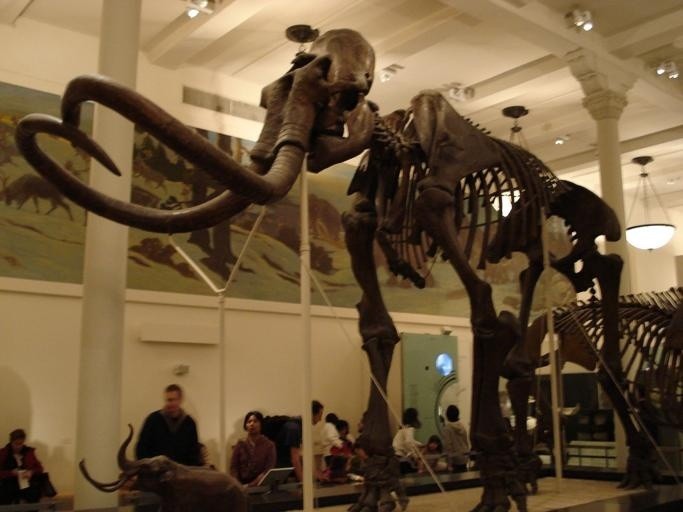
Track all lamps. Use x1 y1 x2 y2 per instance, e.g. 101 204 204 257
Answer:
623 155 676 253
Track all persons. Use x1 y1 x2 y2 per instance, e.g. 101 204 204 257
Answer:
319 404 471 484
0 427 45 505
135 383 203 511
231 412 279 489
272 399 323 482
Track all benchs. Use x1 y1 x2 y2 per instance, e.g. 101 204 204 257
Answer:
239 467 480 506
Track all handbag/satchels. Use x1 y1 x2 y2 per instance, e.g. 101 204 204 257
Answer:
36 472 57 498
